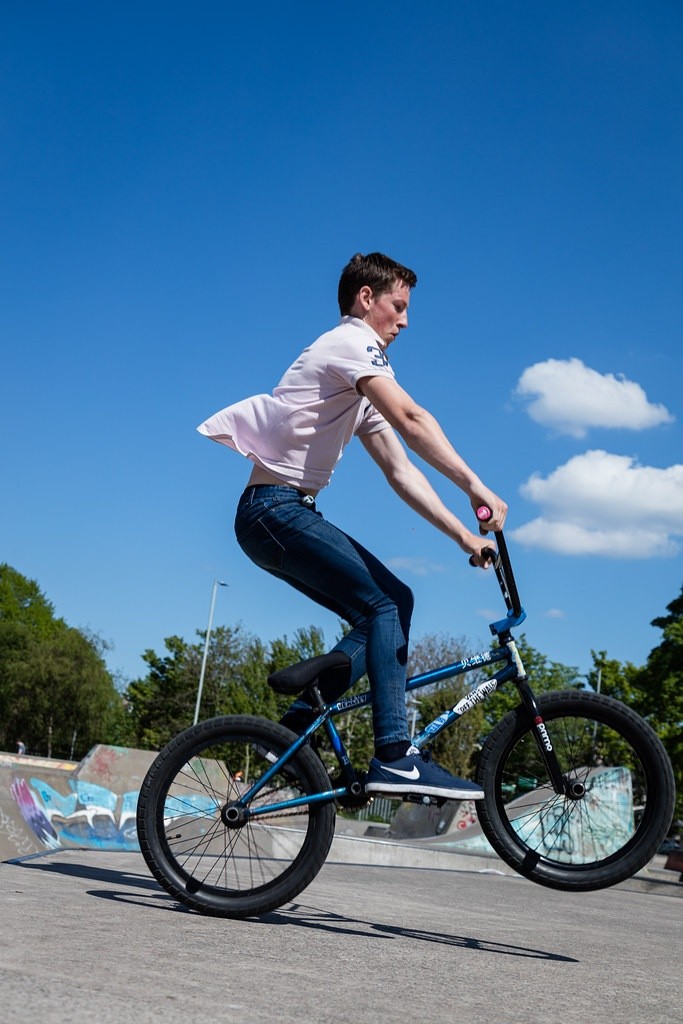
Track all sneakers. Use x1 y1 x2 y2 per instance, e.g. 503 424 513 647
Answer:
365 750 486 799
253 741 307 780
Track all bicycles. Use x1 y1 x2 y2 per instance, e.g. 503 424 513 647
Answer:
135 504 677 920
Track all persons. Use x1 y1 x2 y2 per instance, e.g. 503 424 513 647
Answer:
232 771 244 783
17 741 26 755
194 252 509 801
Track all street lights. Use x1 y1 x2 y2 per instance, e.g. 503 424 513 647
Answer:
194 579 229 726
592 661 607 760
